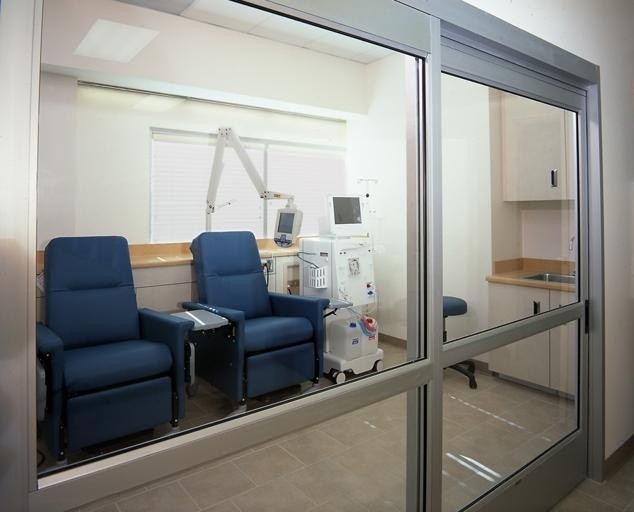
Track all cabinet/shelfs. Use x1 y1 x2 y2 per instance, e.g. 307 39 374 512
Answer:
487 280 580 399
265 255 300 297
500 88 578 201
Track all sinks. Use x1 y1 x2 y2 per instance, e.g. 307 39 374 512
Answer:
522 271 577 286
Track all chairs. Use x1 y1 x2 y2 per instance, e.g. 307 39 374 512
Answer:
35 235 195 464
183 230 330 418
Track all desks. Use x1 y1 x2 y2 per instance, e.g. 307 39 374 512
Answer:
162 310 232 399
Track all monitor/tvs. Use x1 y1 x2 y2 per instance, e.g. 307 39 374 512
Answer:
274 211 303 247
321 194 367 237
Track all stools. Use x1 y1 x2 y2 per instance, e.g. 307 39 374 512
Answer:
440 293 477 389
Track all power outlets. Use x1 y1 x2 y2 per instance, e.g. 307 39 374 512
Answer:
261 259 274 274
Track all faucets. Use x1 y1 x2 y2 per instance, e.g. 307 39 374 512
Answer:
569 237 576 250
